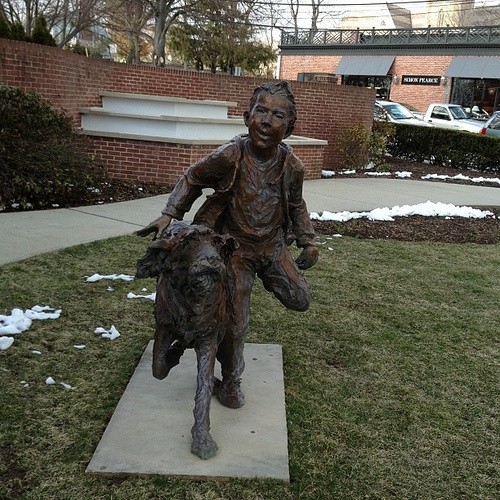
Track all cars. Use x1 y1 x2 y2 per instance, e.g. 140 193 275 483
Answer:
480 111 500 136
374 101 436 128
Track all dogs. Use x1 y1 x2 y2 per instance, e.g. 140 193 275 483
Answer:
134 220 240 461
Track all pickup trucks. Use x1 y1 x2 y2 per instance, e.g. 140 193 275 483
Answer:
410 103 486 133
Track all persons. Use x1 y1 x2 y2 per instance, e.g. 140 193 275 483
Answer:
132 80 320 409
